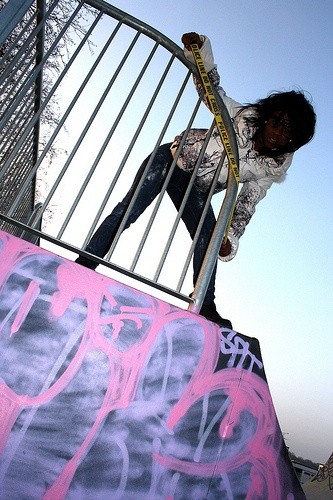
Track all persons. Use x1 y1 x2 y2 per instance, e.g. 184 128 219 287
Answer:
74 32 317 331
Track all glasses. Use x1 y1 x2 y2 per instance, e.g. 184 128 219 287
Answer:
266 115 295 142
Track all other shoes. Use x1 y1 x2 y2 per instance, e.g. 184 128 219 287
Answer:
189 292 233 330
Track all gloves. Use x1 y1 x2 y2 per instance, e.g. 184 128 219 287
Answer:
182 32 203 52
219 237 231 257
